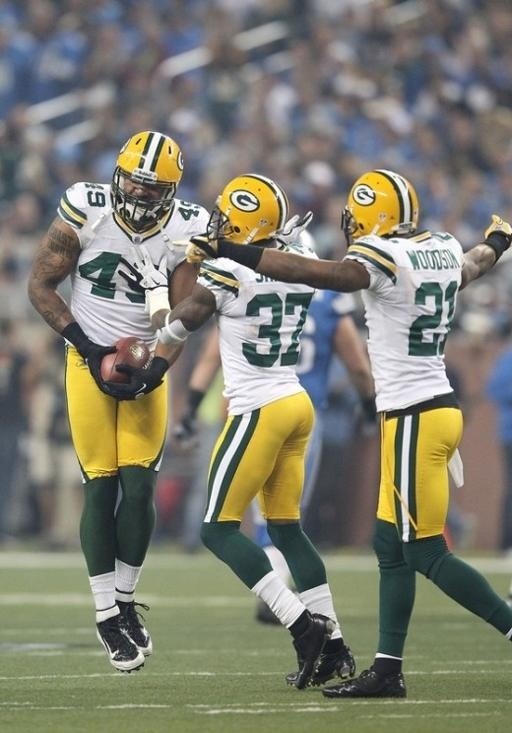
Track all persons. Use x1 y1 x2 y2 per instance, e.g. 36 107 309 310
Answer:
181 164 512 698
138 170 355 688
27 128 213 671
1 1 512 550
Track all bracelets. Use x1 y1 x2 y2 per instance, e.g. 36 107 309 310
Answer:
220 237 267 270
484 233 508 266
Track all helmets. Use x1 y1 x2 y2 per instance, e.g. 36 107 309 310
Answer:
205 174 289 247
341 169 420 240
110 131 185 228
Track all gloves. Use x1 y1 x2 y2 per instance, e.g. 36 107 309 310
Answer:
78 335 117 393
484 214 511 248
184 236 221 264
101 362 163 400
129 244 172 314
274 211 313 253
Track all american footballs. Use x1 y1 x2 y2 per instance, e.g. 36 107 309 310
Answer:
102 338 149 382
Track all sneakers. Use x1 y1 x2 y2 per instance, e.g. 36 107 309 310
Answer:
95 614 145 673
321 668 407 697
284 645 356 686
293 613 337 689
115 600 152 655
